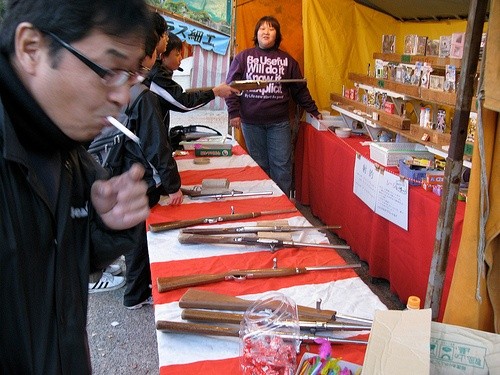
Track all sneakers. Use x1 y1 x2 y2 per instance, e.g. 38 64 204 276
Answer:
104 264 122 275
125 296 154 310
87 272 127 293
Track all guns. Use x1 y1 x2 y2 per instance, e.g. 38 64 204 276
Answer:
147 77 374 345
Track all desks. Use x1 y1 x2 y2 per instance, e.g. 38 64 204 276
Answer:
295 120 466 322
147 135 388 375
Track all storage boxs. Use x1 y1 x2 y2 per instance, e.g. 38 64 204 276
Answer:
371 141 444 184
307 108 353 131
362 309 500 375
194 144 233 156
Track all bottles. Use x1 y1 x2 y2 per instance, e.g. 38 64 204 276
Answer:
420 107 431 128
436 110 446 133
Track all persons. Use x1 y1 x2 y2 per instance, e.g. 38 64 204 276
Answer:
225 15 322 199
0 0 150 375
86 11 240 309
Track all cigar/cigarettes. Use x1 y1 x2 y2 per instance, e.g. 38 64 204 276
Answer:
106 116 139 143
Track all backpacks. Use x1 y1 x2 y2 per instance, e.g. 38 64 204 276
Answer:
87 85 149 174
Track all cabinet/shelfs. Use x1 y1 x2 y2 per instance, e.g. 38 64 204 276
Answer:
330 52 483 161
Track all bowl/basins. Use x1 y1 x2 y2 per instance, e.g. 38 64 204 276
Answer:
335 128 352 138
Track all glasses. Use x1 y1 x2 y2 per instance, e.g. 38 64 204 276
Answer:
48 31 152 89
